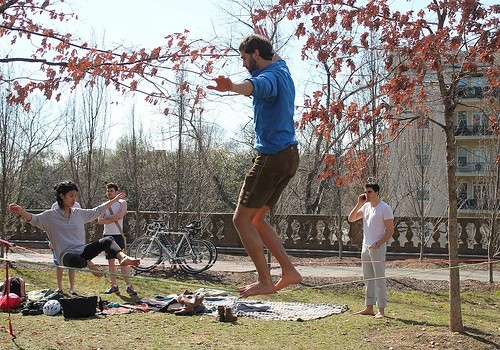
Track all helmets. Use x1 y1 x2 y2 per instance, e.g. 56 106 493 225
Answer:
43 300 61 316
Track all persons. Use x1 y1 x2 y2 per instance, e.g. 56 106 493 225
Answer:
51 200 81 294
10 181 140 277
347 183 394 317
207 24 303 297
97 183 137 295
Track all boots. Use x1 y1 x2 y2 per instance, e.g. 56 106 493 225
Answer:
174 297 196 315
218 306 225 322
225 308 237 322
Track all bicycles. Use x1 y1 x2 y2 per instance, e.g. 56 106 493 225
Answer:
126 220 218 274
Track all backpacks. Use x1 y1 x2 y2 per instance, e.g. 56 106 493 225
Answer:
3 277 25 298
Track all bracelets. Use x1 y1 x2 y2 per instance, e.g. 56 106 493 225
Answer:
376 243 380 247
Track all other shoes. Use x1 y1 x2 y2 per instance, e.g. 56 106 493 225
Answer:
54 290 62 294
70 292 78 296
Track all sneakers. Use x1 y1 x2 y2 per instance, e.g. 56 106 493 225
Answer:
29 302 45 314
126 285 137 295
105 286 119 294
22 302 34 314
